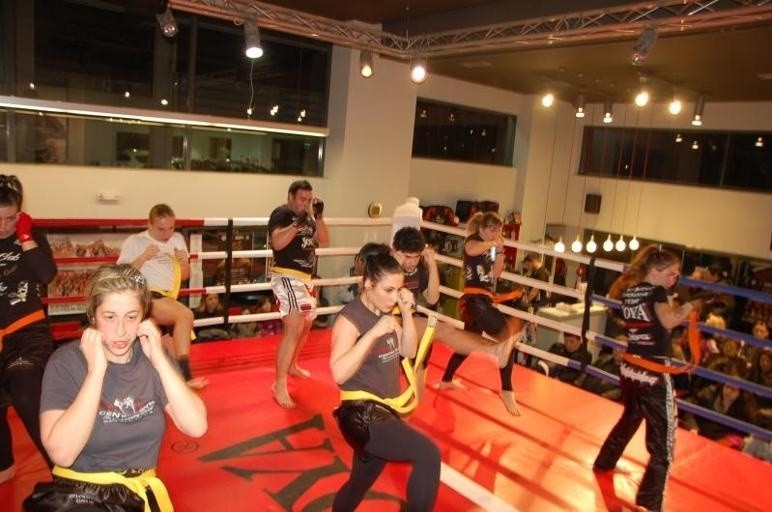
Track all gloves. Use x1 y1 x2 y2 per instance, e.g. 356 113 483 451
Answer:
295 209 308 230
16 212 33 242
312 197 323 219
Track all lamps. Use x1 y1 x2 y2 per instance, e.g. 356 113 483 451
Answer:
541 0 658 255
156 0 772 86
689 93 707 128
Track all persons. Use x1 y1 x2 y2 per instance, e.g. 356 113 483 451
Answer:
438 211 524 419
116 204 210 392
487 251 771 463
0 185 65 483
179 273 284 341
337 225 440 403
268 179 330 409
592 243 705 512
20 264 209 511
316 254 441 512
